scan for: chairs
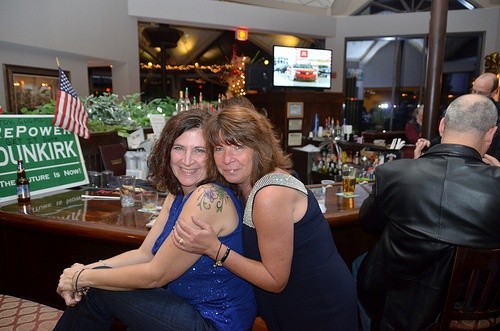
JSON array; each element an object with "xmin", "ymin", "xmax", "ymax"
[{"xmin": 437, "ymin": 246, "xmax": 500, "ymax": 331}]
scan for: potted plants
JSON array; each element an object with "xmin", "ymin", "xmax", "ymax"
[{"xmin": 372, "ymin": 109, "xmax": 387, "ymax": 130}]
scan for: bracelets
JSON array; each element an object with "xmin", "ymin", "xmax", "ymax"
[
  {"xmin": 215, "ymin": 243, "xmax": 222, "ymax": 261},
  {"xmin": 216, "ymin": 248, "xmax": 231, "ymax": 266},
  {"xmin": 76, "ymin": 268, "xmax": 88, "ymax": 295}
]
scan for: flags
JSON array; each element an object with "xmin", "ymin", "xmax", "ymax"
[{"xmin": 52, "ymin": 67, "xmax": 89, "ymax": 139}]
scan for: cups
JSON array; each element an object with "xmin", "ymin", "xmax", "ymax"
[
  {"xmin": 342, "ymin": 167, "xmax": 356, "ymax": 194},
  {"xmin": 140, "ymin": 188, "xmax": 158, "ymax": 219},
  {"xmin": 311, "ymin": 188, "xmax": 325, "ymax": 211},
  {"xmin": 321, "ymin": 180, "xmax": 334, "ymax": 194},
  {"xmin": 119, "ymin": 176, "xmax": 135, "ymax": 207},
  {"xmin": 88, "ymin": 170, "xmax": 113, "ymax": 188}
]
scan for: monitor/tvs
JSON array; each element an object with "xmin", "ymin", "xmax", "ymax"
[{"xmin": 271, "ymin": 45, "xmax": 333, "ymax": 88}]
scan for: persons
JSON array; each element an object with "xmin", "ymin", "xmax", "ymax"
[
  {"xmin": 172, "ymin": 105, "xmax": 360, "ymax": 331},
  {"xmin": 51, "ymin": 109, "xmax": 257, "ymax": 331},
  {"xmin": 403, "ymin": 105, "xmax": 424, "ymax": 159},
  {"xmin": 352, "ymin": 94, "xmax": 500, "ymax": 331},
  {"xmin": 472, "ymin": 73, "xmax": 500, "ymax": 162}
]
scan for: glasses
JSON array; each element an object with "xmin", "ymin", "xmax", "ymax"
[{"xmin": 470, "ymin": 88, "xmax": 495, "ymax": 96}]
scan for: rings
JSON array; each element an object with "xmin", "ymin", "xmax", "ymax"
[{"xmin": 179, "ymin": 239, "xmax": 184, "ymax": 245}]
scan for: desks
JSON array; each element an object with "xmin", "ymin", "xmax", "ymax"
[
  {"xmin": 0, "ymin": 188, "xmax": 166, "ymax": 310},
  {"xmin": 312, "ymin": 183, "xmax": 375, "ymax": 220}
]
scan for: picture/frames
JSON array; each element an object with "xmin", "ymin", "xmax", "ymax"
[{"xmin": 3, "ymin": 64, "xmax": 71, "ymax": 113}]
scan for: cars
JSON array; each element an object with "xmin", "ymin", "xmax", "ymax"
[
  {"xmin": 363, "ymin": 87, "xmax": 419, "ymax": 120},
  {"xmin": 274, "ymin": 63, "xmax": 330, "ymax": 81}
]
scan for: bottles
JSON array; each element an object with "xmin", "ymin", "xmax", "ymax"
[
  {"xmin": 192, "ymin": 93, "xmax": 206, "ymax": 112},
  {"xmin": 335, "ymin": 118, "xmax": 346, "ymax": 138},
  {"xmin": 176, "ymin": 88, "xmax": 191, "ymax": 112},
  {"xmin": 312, "ymin": 144, "xmax": 342, "ymax": 176},
  {"xmin": 17, "ymin": 160, "xmax": 30, "ymax": 203},
  {"xmin": 206, "ymin": 94, "xmax": 226, "ymax": 114},
  {"xmin": 343, "ymin": 150, "xmax": 383, "ymax": 178},
  {"xmin": 324, "ymin": 116, "xmax": 335, "ymax": 139}
]
